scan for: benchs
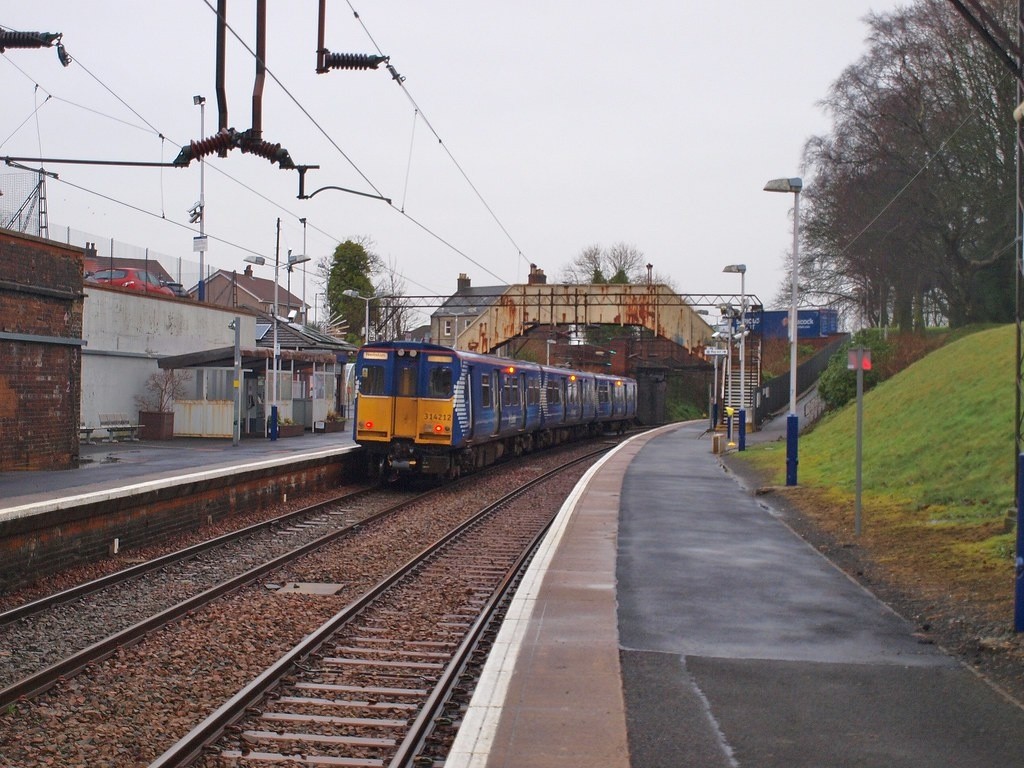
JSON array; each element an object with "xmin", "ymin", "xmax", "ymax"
[
  {"xmin": 80, "ymin": 414, "xmax": 102, "ymax": 445},
  {"xmin": 98, "ymin": 413, "xmax": 146, "ymax": 443}
]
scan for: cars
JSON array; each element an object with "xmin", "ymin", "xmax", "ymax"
[
  {"xmin": 159, "ymin": 280, "xmax": 192, "ymax": 299},
  {"xmin": 88, "ymin": 268, "xmax": 176, "ymax": 297}
]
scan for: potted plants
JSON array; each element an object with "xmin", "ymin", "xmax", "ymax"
[
  {"xmin": 267, "ymin": 417, "xmax": 305, "ymax": 438},
  {"xmin": 314, "ymin": 408, "xmax": 347, "ymax": 433},
  {"xmin": 133, "ymin": 368, "xmax": 194, "ymax": 438}
]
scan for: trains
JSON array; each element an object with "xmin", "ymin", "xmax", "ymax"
[{"xmin": 351, "ymin": 341, "xmax": 638, "ymax": 487}]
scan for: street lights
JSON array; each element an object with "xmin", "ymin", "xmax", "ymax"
[
  {"xmin": 243, "ymin": 254, "xmax": 312, "ymax": 441},
  {"xmin": 722, "ymin": 264, "xmax": 748, "ymax": 452},
  {"xmin": 340, "ymin": 289, "xmax": 395, "ymax": 344},
  {"xmin": 694, "ymin": 308, "xmax": 724, "ymax": 428},
  {"xmin": 762, "ymin": 177, "xmax": 806, "ymax": 488}
]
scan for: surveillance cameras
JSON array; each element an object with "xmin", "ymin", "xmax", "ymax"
[
  {"xmin": 735, "ymin": 331, "xmax": 751, "ymax": 340},
  {"xmin": 288, "ymin": 310, "xmax": 297, "ymax": 322},
  {"xmin": 190, "ymin": 213, "xmax": 200, "ymax": 224},
  {"xmin": 712, "ymin": 332, "xmax": 721, "ymax": 340},
  {"xmin": 187, "ymin": 201, "xmax": 201, "ymax": 213}
]
